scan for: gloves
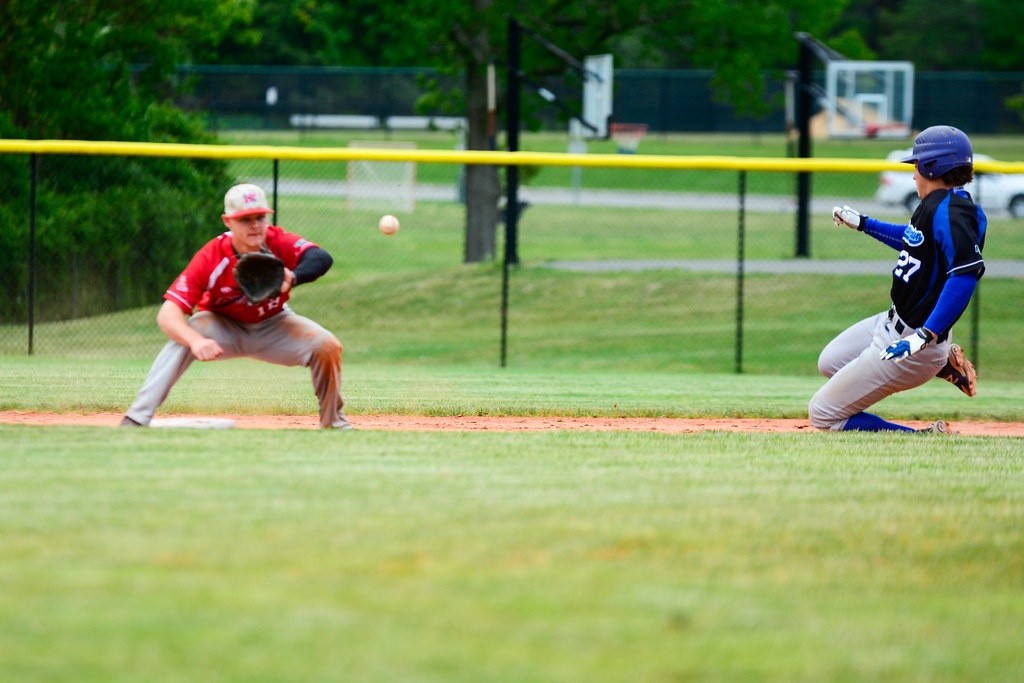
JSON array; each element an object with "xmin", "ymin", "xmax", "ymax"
[
  {"xmin": 880, "ymin": 327, "xmax": 934, "ymax": 364},
  {"xmin": 832, "ymin": 205, "xmax": 868, "ymax": 231}
]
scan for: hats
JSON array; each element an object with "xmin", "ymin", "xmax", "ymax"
[{"xmin": 225, "ymin": 182, "xmax": 274, "ymax": 218}]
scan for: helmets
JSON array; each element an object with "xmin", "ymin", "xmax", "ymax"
[{"xmin": 899, "ymin": 125, "xmax": 973, "ymax": 179}]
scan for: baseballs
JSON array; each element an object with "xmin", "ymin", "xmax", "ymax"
[{"xmin": 379, "ymin": 215, "xmax": 399, "ymax": 235}]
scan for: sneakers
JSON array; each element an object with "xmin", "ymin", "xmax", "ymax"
[
  {"xmin": 930, "ymin": 420, "xmax": 949, "ymax": 435},
  {"xmin": 932, "ymin": 343, "xmax": 977, "ymax": 397}
]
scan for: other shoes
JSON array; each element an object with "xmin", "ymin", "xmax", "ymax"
[
  {"xmin": 324, "ymin": 419, "xmax": 357, "ymax": 429},
  {"xmin": 121, "ymin": 417, "xmax": 137, "ymax": 427}
]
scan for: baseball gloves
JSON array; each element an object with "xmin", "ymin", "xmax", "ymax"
[{"xmin": 233, "ymin": 252, "xmax": 284, "ymax": 302}]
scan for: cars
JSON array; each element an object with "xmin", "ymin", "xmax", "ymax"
[{"xmin": 874, "ymin": 147, "xmax": 1023, "ymax": 222}]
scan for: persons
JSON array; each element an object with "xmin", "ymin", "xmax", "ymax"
[
  {"xmin": 120, "ymin": 183, "xmax": 358, "ymax": 432},
  {"xmin": 808, "ymin": 125, "xmax": 988, "ymax": 434}
]
